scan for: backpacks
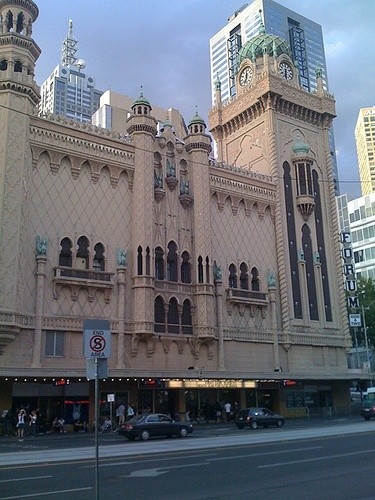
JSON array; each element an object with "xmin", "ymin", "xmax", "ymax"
[{"xmin": 130, "ymin": 406, "xmax": 137, "ymax": 414}]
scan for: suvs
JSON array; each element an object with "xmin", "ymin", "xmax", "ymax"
[{"xmin": 234, "ymin": 407, "xmax": 286, "ymax": 431}]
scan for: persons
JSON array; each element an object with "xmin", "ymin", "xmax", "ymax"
[
  {"xmin": 52, "ymin": 417, "xmax": 65, "ymax": 433},
  {"xmin": 0, "ymin": 407, "xmax": 38, "ymax": 438},
  {"xmin": 115, "ymin": 403, "xmax": 151, "ymax": 426},
  {"xmin": 204, "ymin": 401, "xmax": 240, "ymax": 423},
  {"xmin": 93, "ymin": 416, "xmax": 112, "ymax": 432},
  {"xmin": 74, "ymin": 419, "xmax": 87, "ymax": 432}
]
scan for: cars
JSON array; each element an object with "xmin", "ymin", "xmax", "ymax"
[
  {"xmin": 351, "ymin": 387, "xmax": 375, "ymax": 421},
  {"xmin": 119, "ymin": 413, "xmax": 194, "ymax": 441}
]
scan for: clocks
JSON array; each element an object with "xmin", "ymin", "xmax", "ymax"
[
  {"xmin": 239, "ymin": 64, "xmax": 254, "ymax": 88},
  {"xmin": 280, "ymin": 62, "xmax": 294, "ymax": 80}
]
